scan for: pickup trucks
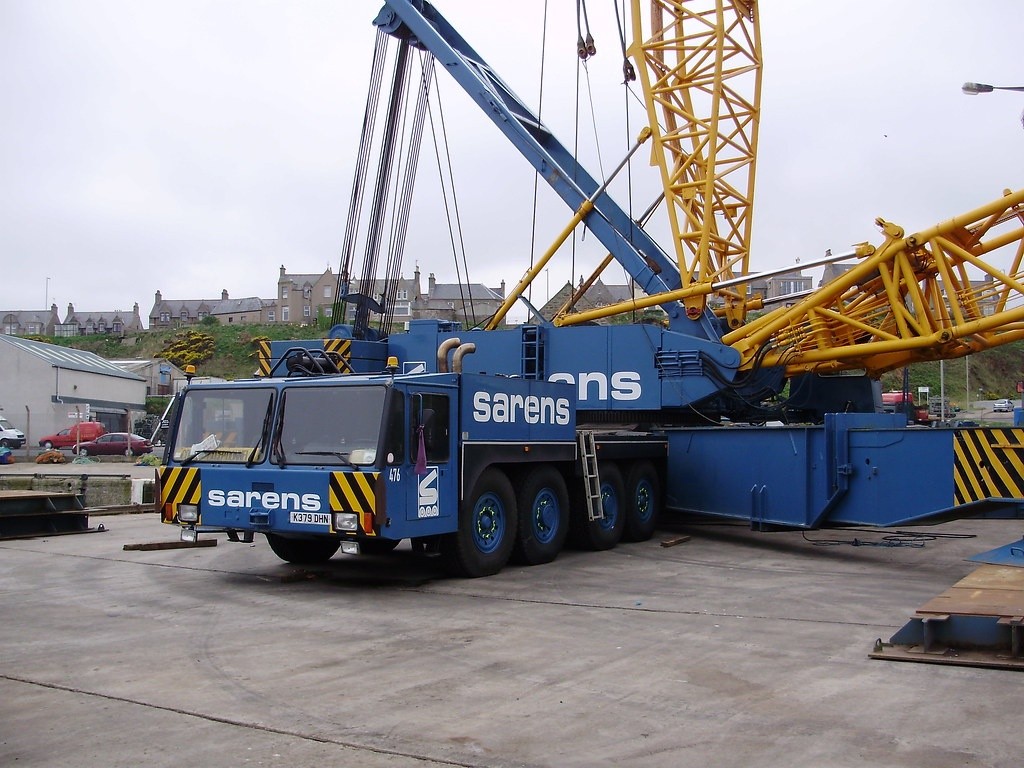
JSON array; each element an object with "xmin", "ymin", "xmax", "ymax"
[{"xmin": 39, "ymin": 422, "xmax": 105, "ymax": 450}]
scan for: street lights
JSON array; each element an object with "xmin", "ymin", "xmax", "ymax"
[
  {"xmin": 545, "ymin": 268, "xmax": 548, "ymax": 302},
  {"xmin": 44, "ymin": 277, "xmax": 51, "ymax": 337}
]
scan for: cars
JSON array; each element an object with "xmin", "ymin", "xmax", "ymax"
[
  {"xmin": 992, "ymin": 398, "xmax": 1015, "ymax": 412},
  {"xmin": 72, "ymin": 432, "xmax": 154, "ymax": 458}
]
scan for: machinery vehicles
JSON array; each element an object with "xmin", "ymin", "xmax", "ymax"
[{"xmin": 142, "ymin": 0, "xmax": 1024, "ymax": 584}]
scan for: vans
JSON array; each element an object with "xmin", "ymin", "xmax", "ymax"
[{"xmin": 0, "ymin": 414, "xmax": 26, "ymax": 449}]
